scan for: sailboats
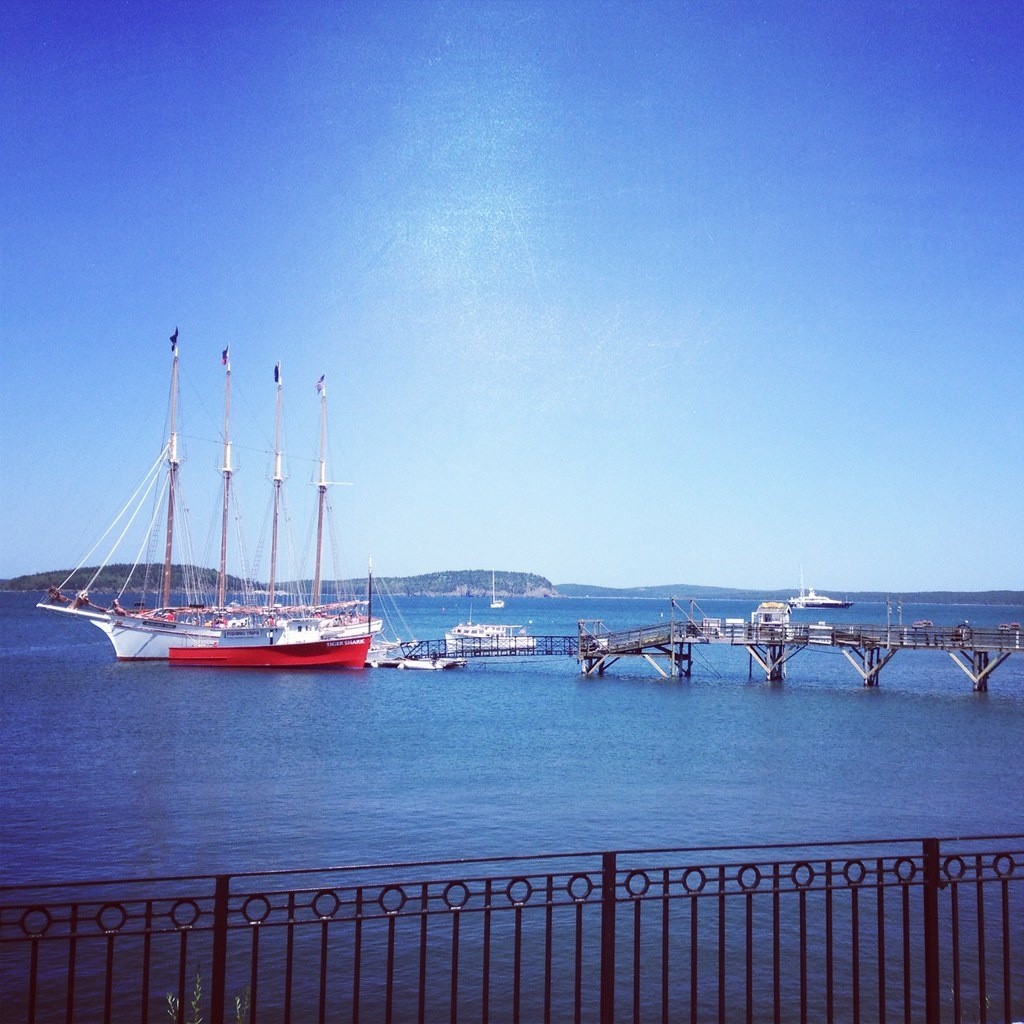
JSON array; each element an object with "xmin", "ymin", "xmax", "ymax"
[
  {"xmin": 359, "ymin": 554, "xmax": 418, "ymax": 667},
  {"xmin": 37, "ymin": 328, "xmax": 383, "ymax": 665},
  {"xmin": 444, "ymin": 603, "xmax": 536, "ymax": 653},
  {"xmin": 787, "ymin": 563, "xmax": 855, "ymax": 609},
  {"xmin": 489, "ymin": 569, "xmax": 505, "ymax": 609}
]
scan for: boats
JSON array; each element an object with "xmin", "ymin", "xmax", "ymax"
[
  {"xmin": 1009, "ymin": 623, "xmax": 1021, "ymax": 630},
  {"xmin": 168, "ymin": 634, "xmax": 372, "ymax": 669},
  {"xmin": 134, "ymin": 597, "xmax": 147, "ymax": 606},
  {"xmin": 923, "ymin": 620, "xmax": 933, "ymax": 627},
  {"xmin": 378, "ymin": 655, "xmax": 467, "ymax": 669},
  {"xmin": 998, "ymin": 624, "xmax": 1011, "ymax": 631},
  {"xmin": 912, "ymin": 621, "xmax": 924, "ymax": 629}
]
